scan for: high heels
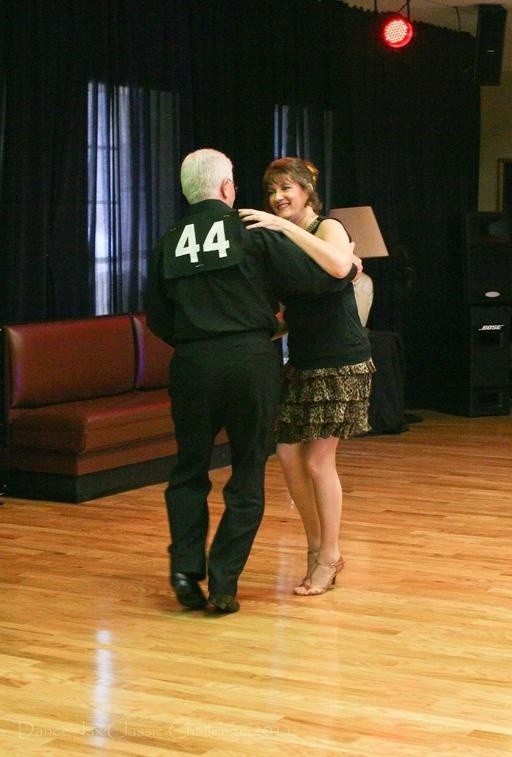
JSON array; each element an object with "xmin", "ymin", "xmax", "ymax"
[{"xmin": 293, "ymin": 551, "xmax": 344, "ymax": 594}]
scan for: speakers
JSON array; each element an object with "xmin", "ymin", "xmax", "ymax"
[{"xmin": 472, "ymin": 3, "xmax": 508, "ymax": 86}]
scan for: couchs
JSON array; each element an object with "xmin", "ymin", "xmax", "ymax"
[{"xmin": 1, "ymin": 311, "xmax": 280, "ymax": 505}]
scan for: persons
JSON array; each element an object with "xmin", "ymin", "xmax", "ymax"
[
  {"xmin": 226, "ymin": 157, "xmax": 359, "ymax": 598},
  {"xmin": 140, "ymin": 147, "xmax": 366, "ymax": 613}
]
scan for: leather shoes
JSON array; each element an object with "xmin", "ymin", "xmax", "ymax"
[
  {"xmin": 206, "ymin": 596, "xmax": 242, "ymax": 612},
  {"xmin": 168, "ymin": 573, "xmax": 208, "ymax": 608}
]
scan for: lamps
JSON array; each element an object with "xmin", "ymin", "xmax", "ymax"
[
  {"xmin": 370, "ymin": 1, "xmax": 416, "ymax": 53},
  {"xmin": 327, "ymin": 204, "xmax": 389, "ymax": 330}
]
